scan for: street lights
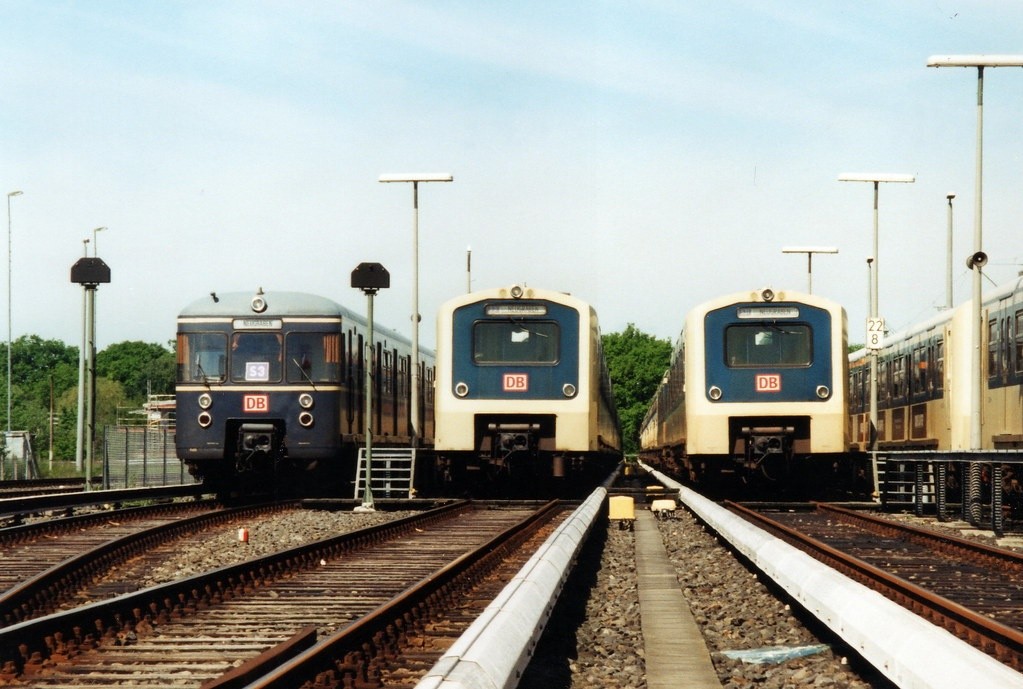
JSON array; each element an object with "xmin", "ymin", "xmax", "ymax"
[
  {"xmin": 86, "ymin": 225, "xmax": 108, "ymax": 490},
  {"xmin": 379, "ymin": 174, "xmax": 454, "ymax": 447},
  {"xmin": 782, "ymin": 247, "xmax": 839, "ymax": 297},
  {"xmin": 839, "ymin": 175, "xmax": 915, "ymax": 451},
  {"xmin": 865, "ymin": 256, "xmax": 874, "ymax": 318},
  {"xmin": 926, "ymin": 51, "xmax": 1023, "ymax": 448},
  {"xmin": 945, "ymin": 193, "xmax": 956, "ymax": 312},
  {"xmin": 7, "ymin": 190, "xmax": 24, "ymax": 433}
]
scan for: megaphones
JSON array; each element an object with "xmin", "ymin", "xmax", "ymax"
[
  {"xmin": 972, "ymin": 252, "xmax": 989, "ymax": 267},
  {"xmin": 966, "ymin": 255, "xmax": 974, "ymax": 269}
]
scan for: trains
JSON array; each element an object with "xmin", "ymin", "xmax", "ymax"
[
  {"xmin": 638, "ymin": 288, "xmax": 845, "ymax": 498},
  {"xmin": 847, "ymin": 272, "xmax": 1023, "ymax": 527},
  {"xmin": 174, "ymin": 286, "xmax": 435, "ymax": 502},
  {"xmin": 434, "ymin": 283, "xmax": 625, "ymax": 503}
]
